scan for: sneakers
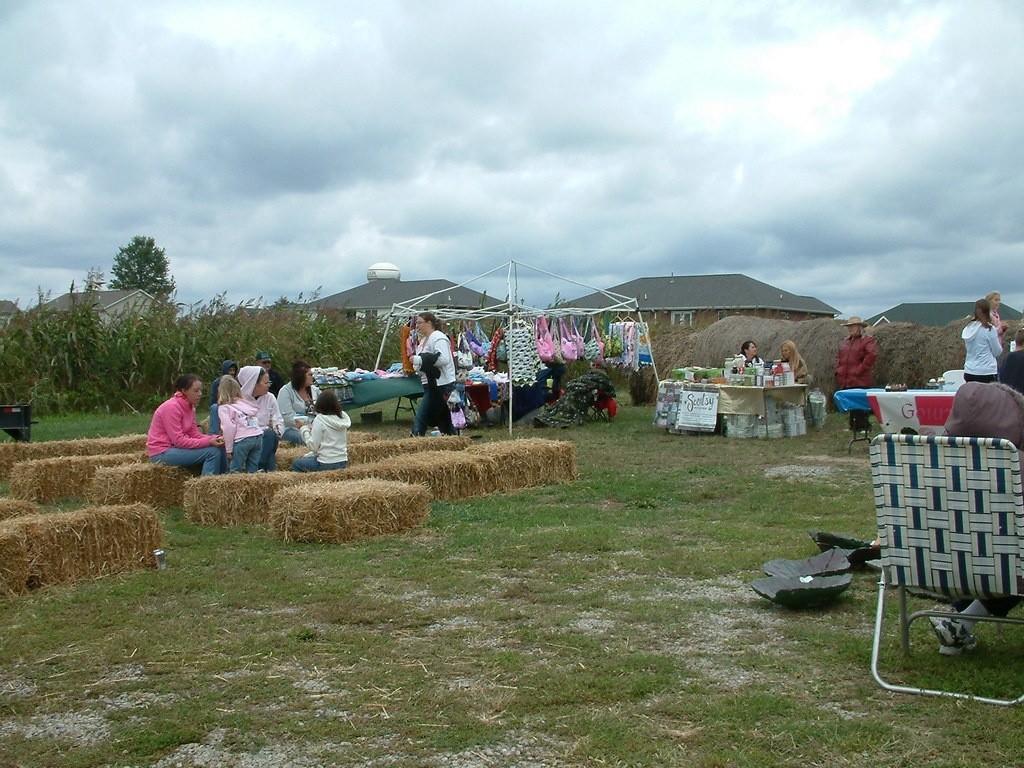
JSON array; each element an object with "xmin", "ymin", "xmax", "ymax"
[
  {"xmin": 928, "ymin": 606, "xmax": 968, "ymax": 647},
  {"xmin": 938, "ymin": 631, "xmax": 978, "ymax": 657}
]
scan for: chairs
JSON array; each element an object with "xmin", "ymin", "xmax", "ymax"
[
  {"xmin": 943, "ymin": 369, "xmax": 966, "ymax": 392},
  {"xmin": 870, "ymin": 433, "xmax": 1024, "ymax": 705},
  {"xmin": 803, "ymin": 374, "xmax": 816, "ymax": 425}
]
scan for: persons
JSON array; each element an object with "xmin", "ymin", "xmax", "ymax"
[
  {"xmin": 209, "ymin": 356, "xmax": 239, "ymax": 435},
  {"xmin": 146, "ymin": 373, "xmax": 230, "ymax": 476},
  {"xmin": 409, "ymin": 313, "xmax": 460, "ymax": 437},
  {"xmin": 276, "ymin": 360, "xmax": 324, "ymax": 444},
  {"xmin": 732, "ymin": 340, "xmax": 767, "ymax": 370},
  {"xmin": 871, "ymin": 383, "xmax": 1024, "ymax": 656},
  {"xmin": 780, "ymin": 338, "xmax": 810, "ymax": 384},
  {"xmin": 291, "ymin": 390, "xmax": 353, "ymax": 472},
  {"xmin": 235, "ymin": 366, "xmax": 286, "ymax": 474},
  {"xmin": 253, "ymin": 351, "xmax": 285, "ymax": 400},
  {"xmin": 834, "ymin": 317, "xmax": 880, "ymax": 433},
  {"xmin": 961, "ymin": 298, "xmax": 1003, "ymax": 385},
  {"xmin": 218, "ymin": 374, "xmax": 263, "ymax": 474},
  {"xmin": 985, "ymin": 291, "xmax": 1010, "ymax": 351},
  {"xmin": 1001, "ymin": 327, "xmax": 1024, "ymax": 395}
]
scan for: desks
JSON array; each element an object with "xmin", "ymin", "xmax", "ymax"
[
  {"xmin": 652, "ymin": 377, "xmax": 808, "ymax": 442},
  {"xmin": 834, "ymin": 387, "xmax": 956, "ymax": 455},
  {"xmin": 314, "ymin": 373, "xmax": 509, "ymax": 438}
]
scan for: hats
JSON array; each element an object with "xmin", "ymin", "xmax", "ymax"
[
  {"xmin": 255, "ymin": 350, "xmax": 272, "ymax": 361},
  {"xmin": 840, "ymin": 316, "xmax": 868, "ymax": 328}
]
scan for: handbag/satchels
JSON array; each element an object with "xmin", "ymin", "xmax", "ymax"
[{"xmin": 447, "ymin": 311, "xmax": 638, "ymax": 370}]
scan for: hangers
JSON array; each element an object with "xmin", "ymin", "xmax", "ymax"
[
  {"xmin": 618, "ymin": 310, "xmax": 637, "ymax": 326},
  {"xmin": 609, "ymin": 309, "xmax": 624, "ymax": 326},
  {"xmin": 503, "ymin": 313, "xmax": 534, "ymax": 330}
]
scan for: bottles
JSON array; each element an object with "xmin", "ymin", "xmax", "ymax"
[
  {"xmin": 810, "ymin": 386, "xmax": 826, "ymax": 426},
  {"xmin": 724, "ymin": 356, "xmax": 794, "ymax": 387}
]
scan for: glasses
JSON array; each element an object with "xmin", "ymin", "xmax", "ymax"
[
  {"xmin": 415, "ymin": 321, "xmax": 423, "ymax": 324},
  {"xmin": 260, "ymin": 360, "xmax": 272, "ymax": 365}
]
